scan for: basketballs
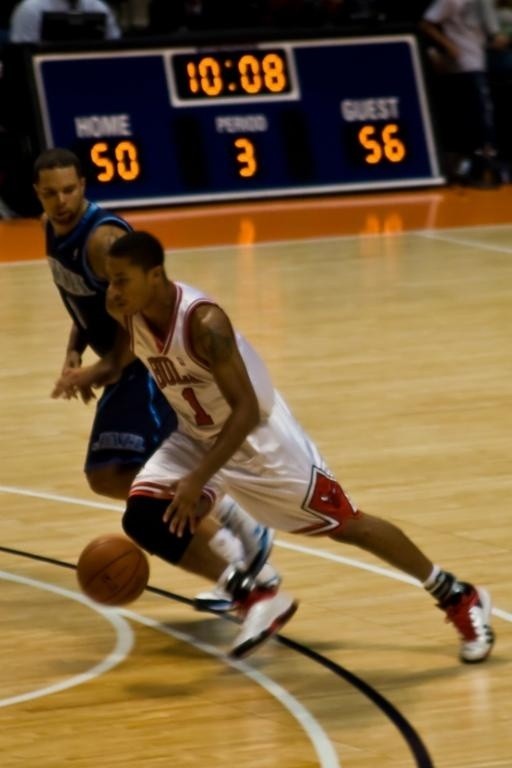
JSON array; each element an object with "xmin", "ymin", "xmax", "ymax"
[{"xmin": 76, "ymin": 536, "xmax": 149, "ymax": 604}]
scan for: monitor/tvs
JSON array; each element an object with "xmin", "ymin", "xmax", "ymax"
[{"xmin": 41, "ymin": 10, "xmax": 108, "ymax": 41}]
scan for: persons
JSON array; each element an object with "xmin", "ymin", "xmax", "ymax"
[
  {"xmin": 104, "ymin": 229, "xmax": 497, "ymax": 663},
  {"xmin": 8, "ymin": 0, "xmax": 123, "ymax": 43},
  {"xmin": 30, "ymin": 150, "xmax": 281, "ymax": 616},
  {"xmin": 420, "ymin": 0, "xmax": 512, "ymax": 157}
]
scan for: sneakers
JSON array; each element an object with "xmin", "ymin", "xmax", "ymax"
[
  {"xmin": 194, "ymin": 523, "xmax": 298, "ymax": 658},
  {"xmin": 435, "ymin": 581, "xmax": 493, "ymax": 662}
]
